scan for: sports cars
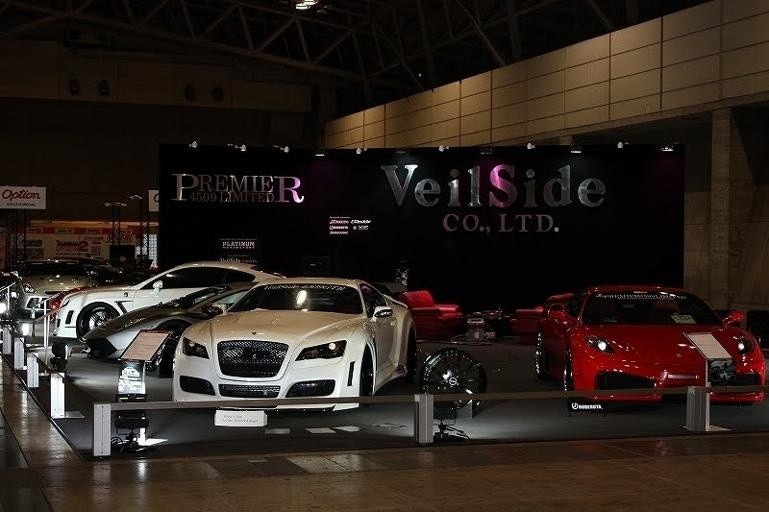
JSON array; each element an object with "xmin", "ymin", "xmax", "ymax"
[
  {"xmin": 1, "ymin": 254, "xmax": 288, "ymax": 365},
  {"xmin": 172, "ymin": 275, "xmax": 420, "ymax": 414},
  {"xmin": 533, "ymin": 275, "xmax": 767, "ymax": 404}
]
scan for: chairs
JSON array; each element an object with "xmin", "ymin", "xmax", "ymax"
[
  {"xmin": 511, "ymin": 293, "xmax": 575, "ymax": 338},
  {"xmin": 399, "ymin": 291, "xmax": 464, "ymax": 341}
]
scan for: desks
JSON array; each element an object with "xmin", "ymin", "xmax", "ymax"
[{"xmin": 457, "ymin": 308, "xmax": 511, "ymax": 337}]
